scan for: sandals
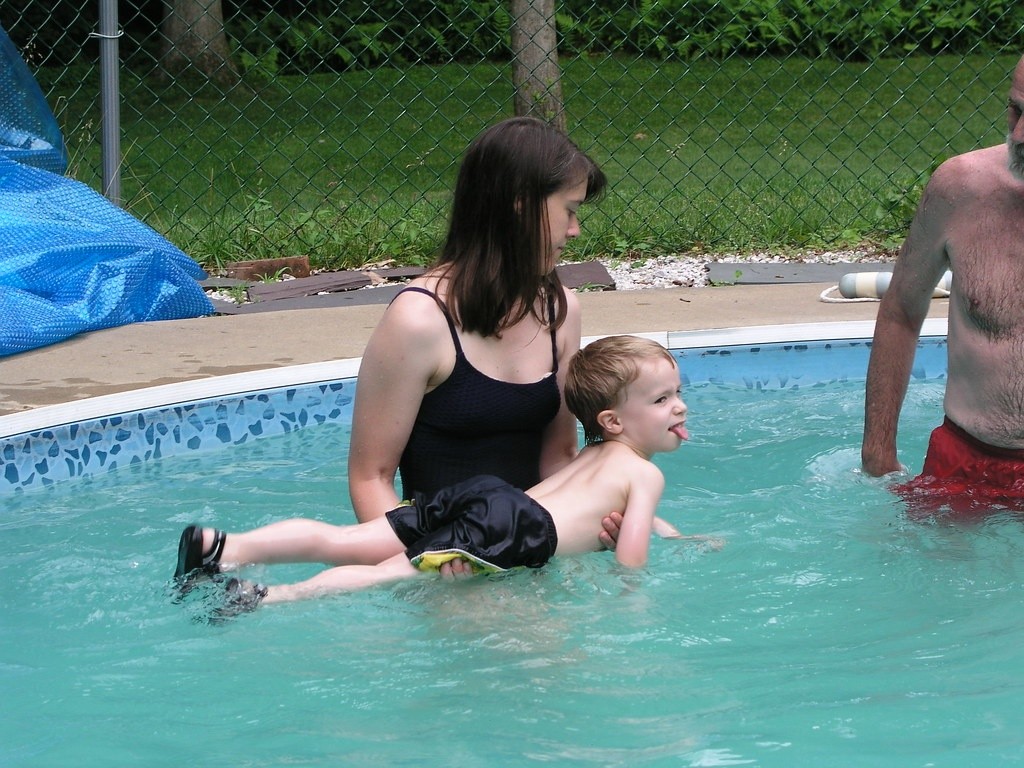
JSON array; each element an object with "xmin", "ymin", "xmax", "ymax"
[
  {"xmin": 171, "ymin": 525, "xmax": 226, "ymax": 581},
  {"xmin": 223, "ymin": 578, "xmax": 269, "ymax": 619}
]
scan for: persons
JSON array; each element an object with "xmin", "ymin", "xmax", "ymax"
[
  {"xmin": 171, "ymin": 336, "xmax": 692, "ymax": 604},
  {"xmin": 861, "ymin": 51, "xmax": 1024, "ymax": 528},
  {"xmin": 346, "ymin": 115, "xmax": 681, "ymax": 584}
]
